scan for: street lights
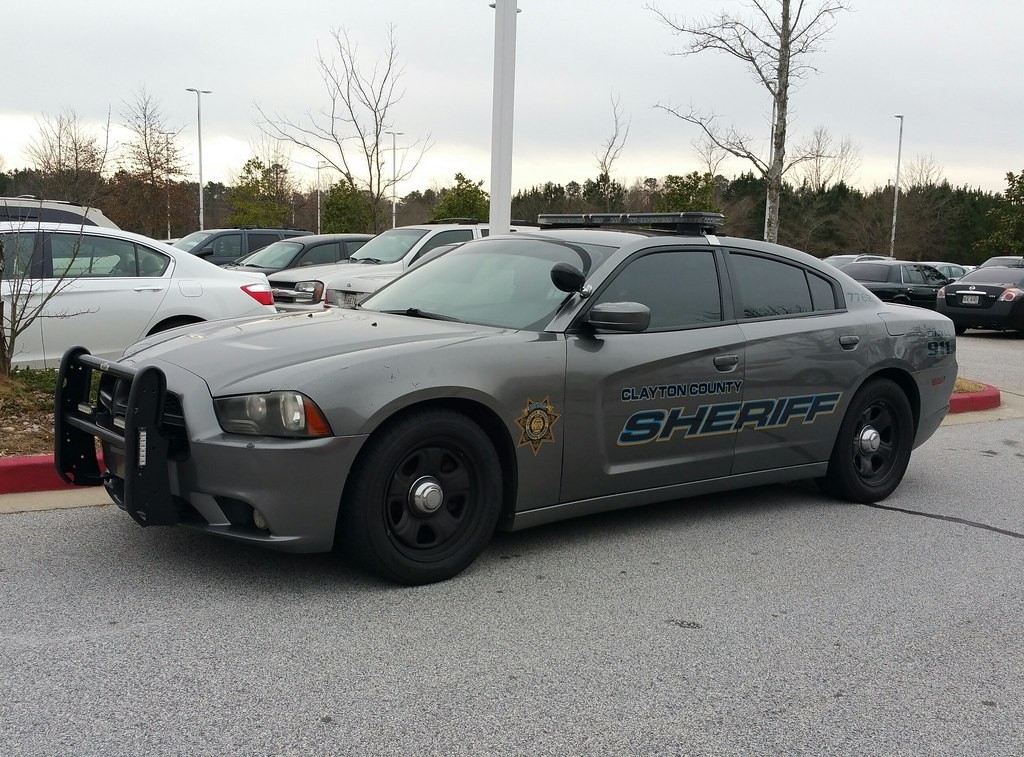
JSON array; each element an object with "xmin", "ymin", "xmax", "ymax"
[
  {"xmin": 889, "ymin": 112, "xmax": 905, "ymax": 257},
  {"xmin": 160, "ymin": 130, "xmax": 177, "ymax": 239},
  {"xmin": 316, "ymin": 161, "xmax": 326, "ymax": 234},
  {"xmin": 386, "ymin": 130, "xmax": 406, "ymax": 228},
  {"xmin": 186, "ymin": 85, "xmax": 212, "ymax": 230}
]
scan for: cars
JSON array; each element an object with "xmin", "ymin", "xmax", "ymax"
[
  {"xmin": 51, "ymin": 206, "xmax": 959, "ymax": 591},
  {"xmin": 220, "ymin": 233, "xmax": 378, "ymax": 278},
  {"xmin": 839, "ymin": 261, "xmax": 956, "ymax": 306},
  {"xmin": 978, "ymin": 256, "xmax": 1024, "ymax": 268},
  {"xmin": 172, "ymin": 225, "xmax": 315, "ymax": 267},
  {"xmin": 923, "ymin": 261, "xmax": 971, "ymax": 282},
  {"xmin": 937, "ymin": 266, "xmax": 1024, "ymax": 336},
  {"xmin": 0, "ymin": 219, "xmax": 278, "ymax": 369}
]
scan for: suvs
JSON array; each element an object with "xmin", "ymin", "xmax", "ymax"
[
  {"xmin": 267, "ymin": 213, "xmax": 543, "ymax": 310},
  {"xmin": 0, "ymin": 194, "xmax": 123, "ymax": 231},
  {"xmin": 823, "ymin": 252, "xmax": 897, "ymax": 271}
]
